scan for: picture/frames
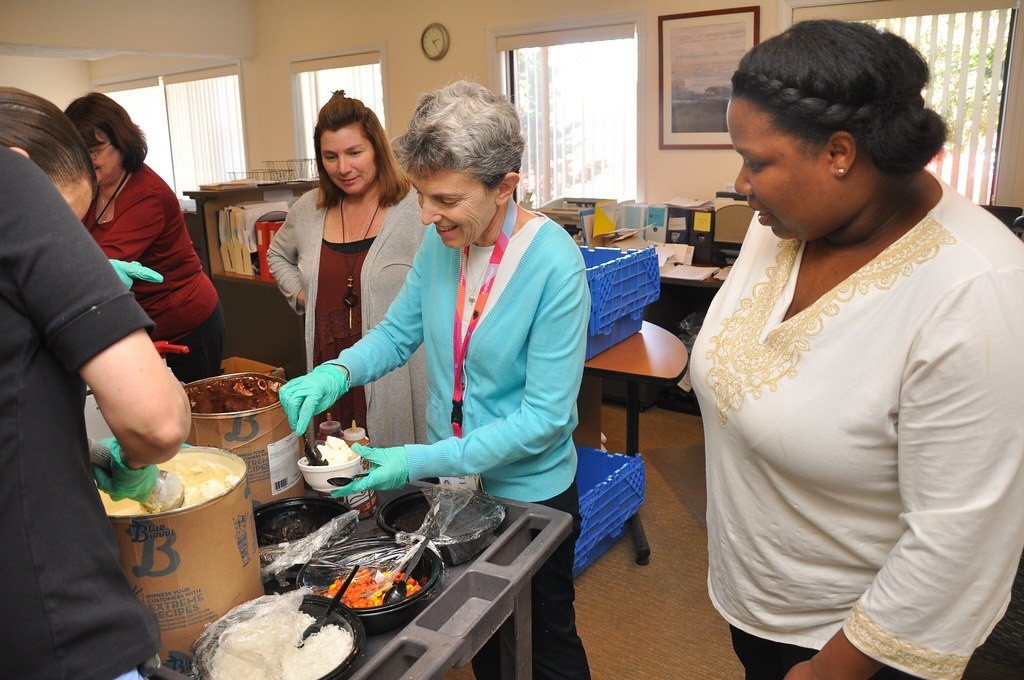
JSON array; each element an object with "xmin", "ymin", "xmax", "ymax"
[{"xmin": 656, "ymin": 4, "xmax": 760, "ymax": 151}]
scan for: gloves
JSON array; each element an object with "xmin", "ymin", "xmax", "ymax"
[
  {"xmin": 279, "ymin": 364, "xmax": 350, "ymax": 437},
  {"xmin": 331, "ymin": 443, "xmax": 408, "ymax": 498},
  {"xmin": 109, "ymin": 259, "xmax": 163, "ymax": 290},
  {"xmin": 92, "ymin": 437, "xmax": 160, "ymax": 504}
]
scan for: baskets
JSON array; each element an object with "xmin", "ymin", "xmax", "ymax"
[{"xmin": 226, "ymin": 158, "xmax": 319, "ymax": 184}]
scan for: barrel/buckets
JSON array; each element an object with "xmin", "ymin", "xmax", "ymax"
[
  {"xmin": 108, "ymin": 446, "xmax": 266, "ymax": 677},
  {"xmin": 183, "ymin": 372, "xmax": 305, "ymax": 504}
]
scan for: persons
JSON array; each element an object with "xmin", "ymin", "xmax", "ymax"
[
  {"xmin": 61, "ymin": 90, "xmax": 226, "ymax": 382},
  {"xmin": 0, "ymin": 84, "xmax": 191, "ymax": 680},
  {"xmin": 277, "ymin": 80, "xmax": 594, "ymax": 680},
  {"xmin": 689, "ymin": 18, "xmax": 1024, "ymax": 680},
  {"xmin": 266, "ymin": 89, "xmax": 435, "ymax": 448}
]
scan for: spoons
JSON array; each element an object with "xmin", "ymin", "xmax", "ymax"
[
  {"xmin": 383, "ymin": 534, "xmax": 430, "ymax": 606},
  {"xmin": 296, "ymin": 564, "xmax": 360, "ymax": 648},
  {"xmin": 327, "ymin": 473, "xmax": 370, "ymax": 488},
  {"xmin": 349, "ymin": 487, "xmax": 350, "ymax": 488}
]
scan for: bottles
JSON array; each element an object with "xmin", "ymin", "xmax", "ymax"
[{"xmin": 316, "ymin": 413, "xmax": 378, "ymax": 520}]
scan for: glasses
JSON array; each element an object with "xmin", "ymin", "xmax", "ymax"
[{"xmin": 89, "ymin": 142, "xmax": 112, "ymax": 160}]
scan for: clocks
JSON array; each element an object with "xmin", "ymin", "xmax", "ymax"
[{"xmin": 420, "ymin": 22, "xmax": 450, "ymax": 61}]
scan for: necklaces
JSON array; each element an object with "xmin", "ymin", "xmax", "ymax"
[
  {"xmin": 84, "ymin": 170, "xmax": 129, "ymax": 233},
  {"xmin": 465, "ymin": 259, "xmax": 488, "ymax": 305},
  {"xmin": 100, "ymin": 199, "xmax": 109, "ymax": 223},
  {"xmin": 340, "ymin": 195, "xmax": 379, "ymax": 329}
]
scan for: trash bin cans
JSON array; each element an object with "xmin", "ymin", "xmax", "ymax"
[{"xmin": 670, "ymin": 310, "xmax": 710, "ymax": 414}]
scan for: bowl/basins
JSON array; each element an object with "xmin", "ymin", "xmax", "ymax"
[
  {"xmin": 296, "ymin": 536, "xmax": 442, "ymax": 635},
  {"xmin": 296, "ymin": 454, "xmax": 361, "ymax": 493},
  {"xmin": 375, "ymin": 486, "xmax": 506, "ymax": 568},
  {"xmin": 252, "ymin": 495, "xmax": 359, "ymax": 582},
  {"xmin": 191, "ymin": 595, "xmax": 367, "ymax": 679}
]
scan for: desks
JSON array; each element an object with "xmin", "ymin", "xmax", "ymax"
[
  {"xmin": 184, "ymin": 178, "xmax": 320, "ymax": 382},
  {"xmin": 575, "ymin": 322, "xmax": 689, "ymax": 567},
  {"xmin": 632, "ymin": 258, "xmax": 732, "ymax": 413}
]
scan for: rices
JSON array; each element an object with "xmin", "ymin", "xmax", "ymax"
[
  {"xmin": 208, "ymin": 610, "xmax": 353, "ymax": 680},
  {"xmin": 302, "ymin": 435, "xmax": 355, "ymax": 468}
]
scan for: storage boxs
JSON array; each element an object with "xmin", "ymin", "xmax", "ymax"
[
  {"xmin": 568, "ymin": 447, "xmax": 644, "ymax": 578},
  {"xmin": 580, "ymin": 246, "xmax": 660, "ymax": 362}
]
